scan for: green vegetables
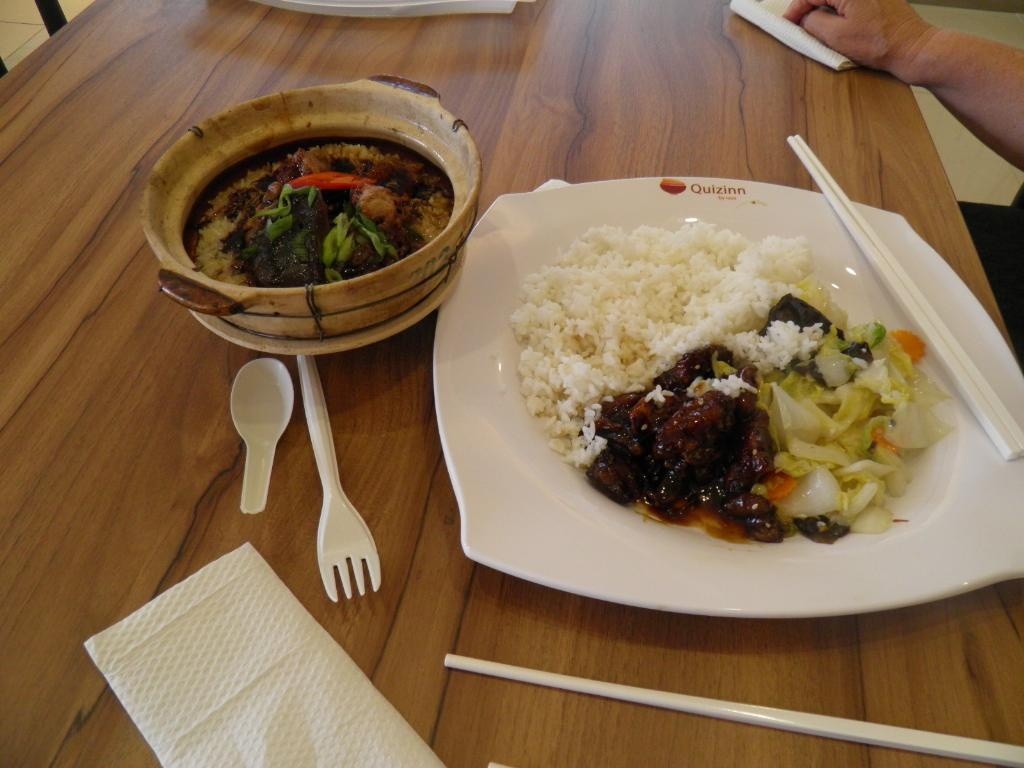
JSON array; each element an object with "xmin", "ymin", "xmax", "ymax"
[{"xmin": 246, "ymin": 184, "xmax": 398, "ymax": 283}]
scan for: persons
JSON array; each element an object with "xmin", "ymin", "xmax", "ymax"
[{"xmin": 780, "ymin": 0, "xmax": 1023, "ymax": 375}]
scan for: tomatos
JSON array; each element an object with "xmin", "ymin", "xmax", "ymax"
[{"xmin": 291, "ymin": 170, "xmax": 376, "ymax": 191}]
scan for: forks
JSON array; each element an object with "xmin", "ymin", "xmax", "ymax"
[{"xmin": 289, "ymin": 355, "xmax": 382, "ymax": 604}]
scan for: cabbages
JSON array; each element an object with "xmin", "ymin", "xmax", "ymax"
[{"xmin": 759, "ymin": 321, "xmax": 958, "ymax": 536}]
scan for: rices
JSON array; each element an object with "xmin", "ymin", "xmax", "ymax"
[{"xmin": 510, "ymin": 221, "xmax": 814, "ymax": 465}]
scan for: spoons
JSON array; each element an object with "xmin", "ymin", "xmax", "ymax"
[{"xmin": 227, "ymin": 358, "xmax": 294, "ymax": 515}]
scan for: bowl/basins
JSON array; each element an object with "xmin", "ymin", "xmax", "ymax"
[{"xmin": 144, "ymin": 74, "xmax": 480, "ymax": 356}]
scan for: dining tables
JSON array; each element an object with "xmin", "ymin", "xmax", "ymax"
[{"xmin": 1, "ymin": 2, "xmax": 1024, "ymax": 768}]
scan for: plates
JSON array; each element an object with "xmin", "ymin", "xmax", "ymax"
[{"xmin": 434, "ymin": 168, "xmax": 1023, "ymax": 625}]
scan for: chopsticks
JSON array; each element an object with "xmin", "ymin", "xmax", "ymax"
[{"xmin": 787, "ymin": 130, "xmax": 1023, "ymax": 464}]
